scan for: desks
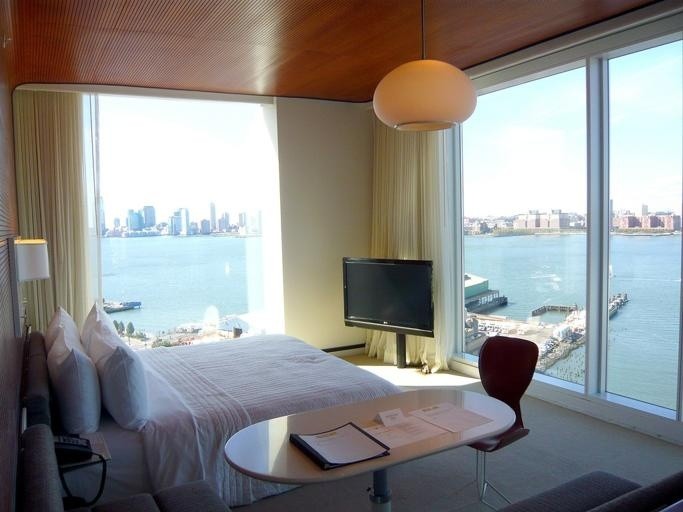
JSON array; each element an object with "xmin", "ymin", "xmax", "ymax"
[{"xmin": 222, "ymin": 387, "xmax": 517, "ymax": 512}]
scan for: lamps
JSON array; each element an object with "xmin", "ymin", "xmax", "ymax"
[
  {"xmin": 14, "ymin": 238, "xmax": 50, "ymax": 281},
  {"xmin": 372, "ymin": 0, "xmax": 477, "ymax": 133}
]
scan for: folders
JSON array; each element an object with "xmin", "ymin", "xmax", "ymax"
[{"xmin": 288, "ymin": 422, "xmax": 391, "ymax": 470}]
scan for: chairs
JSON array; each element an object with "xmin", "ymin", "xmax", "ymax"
[
  {"xmin": 498, "ymin": 470, "xmax": 683, "ymax": 512},
  {"xmin": 462, "ymin": 335, "xmax": 538, "ymax": 512},
  {"xmin": 23, "ymin": 424, "xmax": 230, "ymax": 508}
]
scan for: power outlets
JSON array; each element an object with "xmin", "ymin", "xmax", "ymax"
[{"xmin": 20, "ymin": 408, "xmax": 27, "ymax": 434}]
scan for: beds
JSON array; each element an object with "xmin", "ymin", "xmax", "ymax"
[{"xmin": 22, "ymin": 331, "xmax": 402, "ymax": 509}]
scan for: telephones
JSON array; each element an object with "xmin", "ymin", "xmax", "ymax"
[{"xmin": 51, "ymin": 429, "xmax": 91, "ymax": 463}]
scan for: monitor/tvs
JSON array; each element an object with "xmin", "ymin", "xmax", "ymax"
[{"xmin": 342, "ymin": 257, "xmax": 437, "ymax": 338}]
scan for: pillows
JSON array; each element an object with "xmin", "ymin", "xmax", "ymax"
[
  {"xmin": 46, "ymin": 330, "xmax": 100, "ymax": 434},
  {"xmin": 81, "ymin": 300, "xmax": 119, "ymax": 346},
  {"xmin": 45, "ymin": 307, "xmax": 81, "ymax": 349},
  {"xmin": 88, "ymin": 321, "xmax": 150, "ymax": 432}
]
